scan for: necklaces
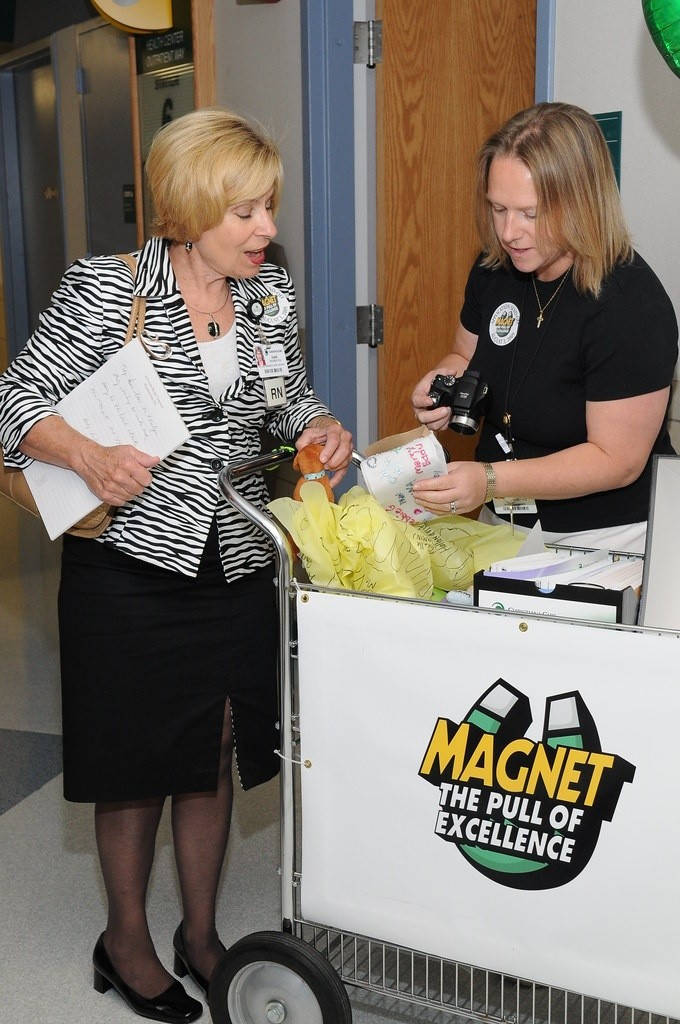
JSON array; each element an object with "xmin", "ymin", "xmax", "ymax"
[
  {"xmin": 185, "ymin": 284, "xmax": 229, "ymax": 337},
  {"xmin": 532, "ymin": 258, "xmax": 575, "ymax": 328}
]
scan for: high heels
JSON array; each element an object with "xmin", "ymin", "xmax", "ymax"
[
  {"xmin": 93, "ymin": 926, "xmax": 203, "ymax": 1024},
  {"xmin": 172, "ymin": 919, "xmax": 227, "ymax": 1006}
]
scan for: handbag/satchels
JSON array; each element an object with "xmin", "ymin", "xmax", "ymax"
[{"xmin": 0, "ymin": 253, "xmax": 146, "ymax": 538}]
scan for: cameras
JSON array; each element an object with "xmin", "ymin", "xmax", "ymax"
[{"xmin": 424, "ymin": 369, "xmax": 495, "ymax": 436}]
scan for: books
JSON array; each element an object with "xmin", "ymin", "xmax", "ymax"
[{"xmin": 488, "ymin": 553, "xmax": 644, "ymax": 597}]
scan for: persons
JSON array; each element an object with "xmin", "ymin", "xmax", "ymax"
[
  {"xmin": 0, "ymin": 110, "xmax": 354, "ymax": 1024},
  {"xmin": 409, "ymin": 102, "xmax": 679, "ymax": 559}
]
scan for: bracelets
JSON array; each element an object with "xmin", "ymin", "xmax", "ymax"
[
  {"xmin": 482, "ymin": 461, "xmax": 495, "ymax": 503},
  {"xmin": 316, "ymin": 417, "xmax": 341, "ymax": 427}
]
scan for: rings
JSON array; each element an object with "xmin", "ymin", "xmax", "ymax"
[
  {"xmin": 415, "ymin": 408, "xmax": 421, "ymax": 420},
  {"xmin": 451, "ymin": 502, "xmax": 455, "ymax": 513}
]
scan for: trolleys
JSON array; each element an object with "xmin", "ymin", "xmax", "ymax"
[{"xmin": 205, "ymin": 446, "xmax": 680, "ymax": 1024}]
